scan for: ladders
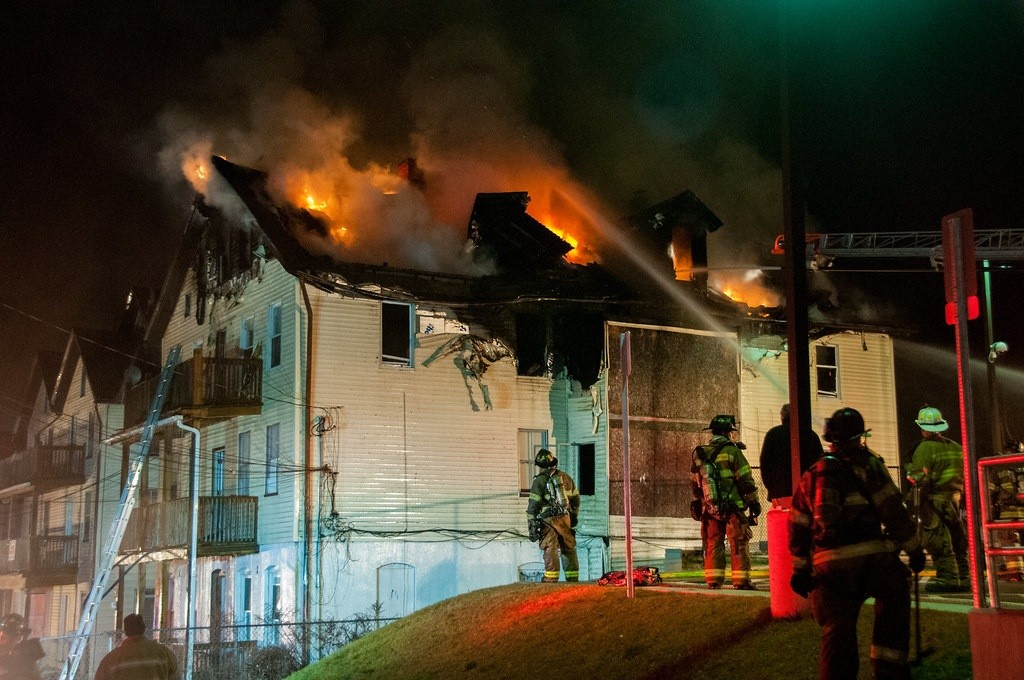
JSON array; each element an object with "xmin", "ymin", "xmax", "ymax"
[{"xmin": 58, "ymin": 344, "xmax": 183, "ymax": 680}]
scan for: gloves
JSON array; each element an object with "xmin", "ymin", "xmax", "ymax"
[
  {"xmin": 747, "ymin": 500, "xmax": 761, "ymax": 518},
  {"xmin": 790, "ymin": 568, "xmax": 814, "ymax": 598},
  {"xmin": 570, "ymin": 513, "xmax": 578, "ymax": 527},
  {"xmin": 907, "ymin": 544, "xmax": 926, "ymax": 574}
]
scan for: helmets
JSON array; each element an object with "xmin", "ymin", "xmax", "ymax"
[
  {"xmin": 823, "ymin": 407, "xmax": 865, "ymax": 442},
  {"xmin": 915, "ymin": 406, "xmax": 949, "ymax": 432},
  {"xmin": 535, "ymin": 449, "xmax": 557, "ymax": 468},
  {"xmin": 0, "ymin": 613, "xmax": 31, "ymax": 636},
  {"xmin": 703, "ymin": 416, "xmax": 738, "ymax": 430}
]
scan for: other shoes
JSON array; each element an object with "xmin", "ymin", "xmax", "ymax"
[
  {"xmin": 542, "ymin": 577, "xmax": 558, "ymax": 583},
  {"xmin": 871, "ymin": 660, "xmax": 912, "ymax": 680},
  {"xmin": 733, "ymin": 581, "xmax": 758, "ymax": 590},
  {"xmin": 925, "ymin": 583, "xmax": 970, "ymax": 594},
  {"xmin": 708, "ymin": 583, "xmax": 721, "ymax": 589},
  {"xmin": 566, "ymin": 578, "xmax": 578, "ymax": 582}
]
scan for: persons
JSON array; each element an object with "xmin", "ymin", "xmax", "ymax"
[
  {"xmin": 787, "ymin": 407, "xmax": 928, "ymax": 680},
  {"xmin": 93, "ymin": 613, "xmax": 181, "ymax": 680},
  {"xmin": 757, "ymin": 404, "xmax": 826, "ymax": 503},
  {"xmin": 686, "ymin": 415, "xmax": 763, "ymax": 590},
  {"xmin": 526, "ymin": 449, "xmax": 582, "ymax": 583},
  {"xmin": 0, "ymin": 612, "xmax": 46, "ymax": 680},
  {"xmin": 907, "ymin": 402, "xmax": 974, "ymax": 595}
]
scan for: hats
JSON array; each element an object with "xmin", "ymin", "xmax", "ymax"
[{"xmin": 123, "ymin": 612, "xmax": 145, "ymax": 636}]
